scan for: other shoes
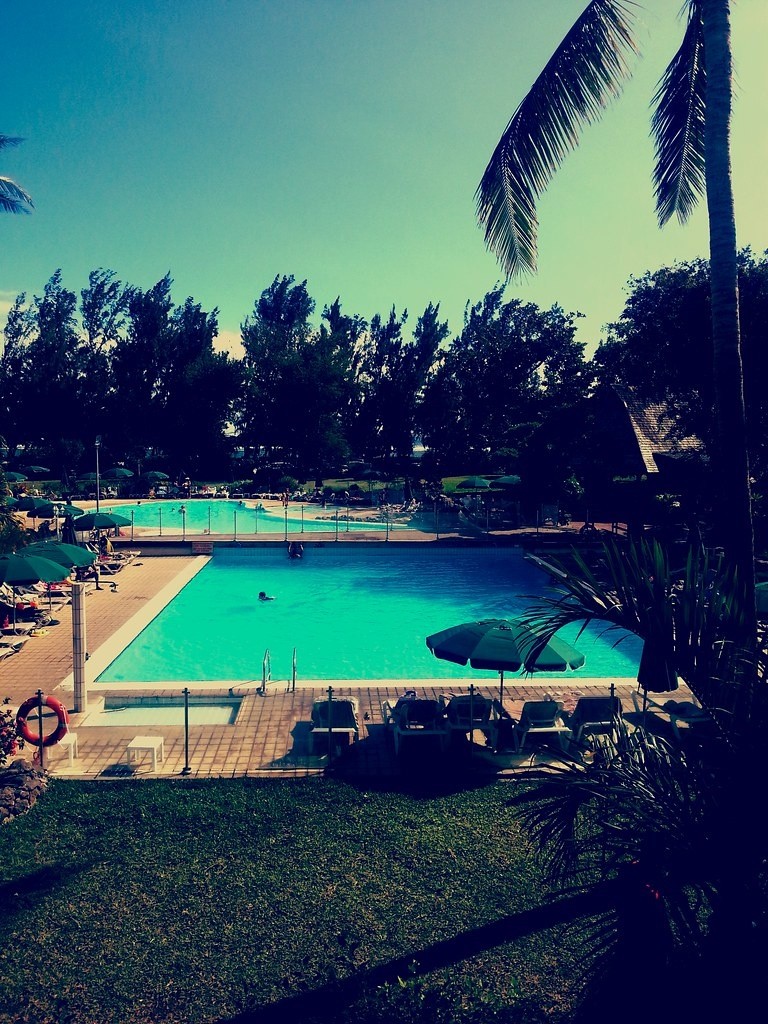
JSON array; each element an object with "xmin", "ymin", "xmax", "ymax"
[{"xmin": 96, "ymin": 586, "xmax": 103, "ymax": 590}]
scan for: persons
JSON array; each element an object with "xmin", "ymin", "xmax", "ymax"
[
  {"xmin": 90, "ymin": 527, "xmax": 100, "ymax": 542},
  {"xmin": 18, "ymin": 485, "xmax": 27, "ymax": 494},
  {"xmin": 280, "ymin": 485, "xmax": 504, "ymax": 522},
  {"xmin": 202, "ymin": 484, "xmax": 208, "ymax": 497},
  {"xmin": 217, "ymin": 485, "xmax": 230, "ymax": 498},
  {"xmin": 173, "ymin": 479, "xmax": 180, "ymax": 499},
  {"xmin": 0, "ymin": 601, "xmax": 44, "ymax": 624},
  {"xmin": 100, "ymin": 535, "xmax": 114, "ymax": 554},
  {"xmin": 148, "ymin": 489, "xmax": 156, "ymax": 498},
  {"xmin": 181, "ymin": 477, "xmax": 192, "ymax": 498},
  {"xmin": 12, "ymin": 485, "xmax": 22, "ymax": 512},
  {"xmin": 74, "ymin": 563, "xmax": 104, "ymax": 590},
  {"xmin": 25, "ymin": 487, "xmax": 31, "ymax": 494},
  {"xmin": 38, "ymin": 520, "xmax": 54, "ymax": 539}
]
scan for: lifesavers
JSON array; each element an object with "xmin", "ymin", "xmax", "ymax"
[{"xmin": 15, "ymin": 697, "xmax": 70, "ymax": 747}]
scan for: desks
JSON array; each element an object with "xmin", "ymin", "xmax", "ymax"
[
  {"xmin": 125, "ymin": 735, "xmax": 164, "ymax": 773},
  {"xmin": 43, "ymin": 732, "xmax": 78, "ymax": 767}
]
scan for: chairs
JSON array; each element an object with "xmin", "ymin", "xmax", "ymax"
[
  {"xmin": 548, "ymin": 690, "xmax": 623, "ymax": 754},
  {"xmin": 632, "ymin": 689, "xmax": 713, "ymax": 742},
  {"xmin": 383, "ymin": 690, "xmax": 447, "ymax": 756},
  {"xmin": 439, "ymin": 692, "xmax": 497, "ymax": 754},
  {"xmin": 0, "ymin": 543, "xmax": 143, "ymax": 660},
  {"xmin": 217, "ymin": 491, "xmax": 369, "ymax": 504},
  {"xmin": 497, "ymin": 697, "xmax": 573, "ymax": 754},
  {"xmin": 309, "ymin": 695, "xmax": 362, "ymax": 752}
]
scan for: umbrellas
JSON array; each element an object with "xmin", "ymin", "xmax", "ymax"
[
  {"xmin": 426, "ymin": 618, "xmax": 585, "ymax": 720},
  {"xmin": 456, "ymin": 474, "xmax": 521, "ymax": 509},
  {"xmin": 0, "ymin": 464, "xmax": 169, "ymax": 632}
]
